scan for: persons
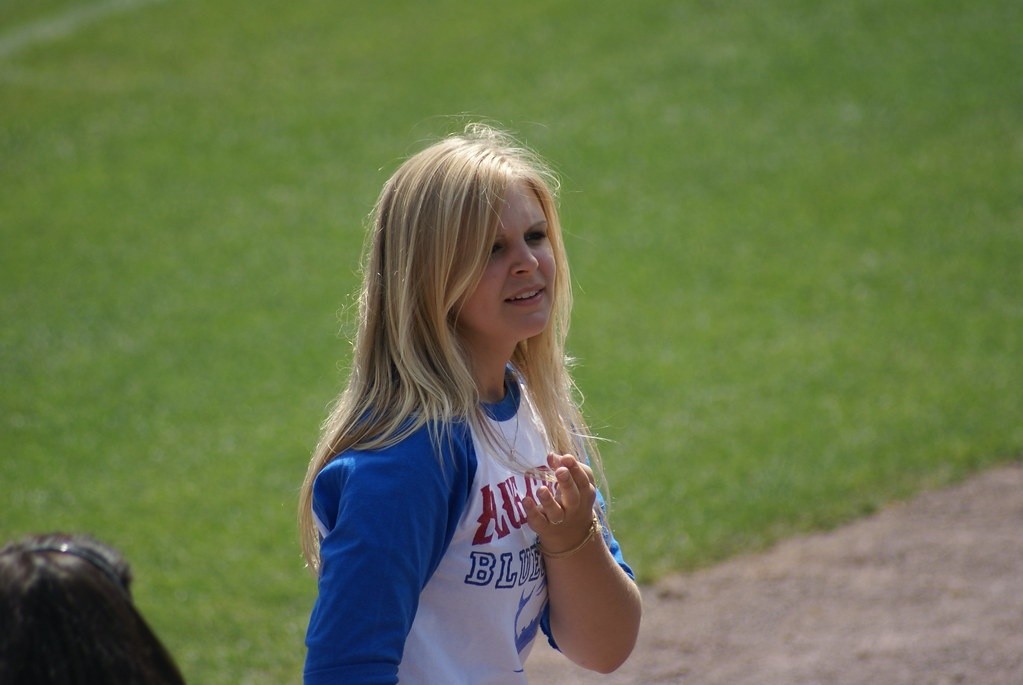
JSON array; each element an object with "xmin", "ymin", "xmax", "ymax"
[
  {"xmin": 1, "ymin": 534, "xmax": 186, "ymax": 685},
  {"xmin": 303, "ymin": 121, "xmax": 641, "ymax": 684}
]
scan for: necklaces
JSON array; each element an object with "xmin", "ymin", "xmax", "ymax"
[{"xmin": 482, "ymin": 379, "xmax": 519, "ymax": 455}]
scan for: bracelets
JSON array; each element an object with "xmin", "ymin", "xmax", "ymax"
[{"xmin": 536, "ymin": 514, "xmax": 597, "ymax": 558}]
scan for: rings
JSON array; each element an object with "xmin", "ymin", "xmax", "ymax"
[{"xmin": 549, "ymin": 520, "xmax": 563, "ymax": 525}]
